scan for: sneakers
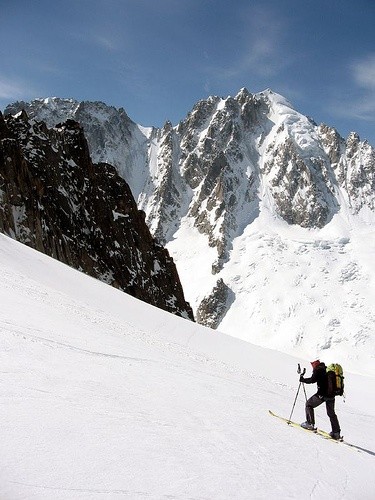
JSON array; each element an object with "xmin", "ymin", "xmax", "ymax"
[
  {"xmin": 301, "ymin": 422, "xmax": 314, "ymax": 430},
  {"xmin": 330, "ymin": 432, "xmax": 340, "ymax": 439}
]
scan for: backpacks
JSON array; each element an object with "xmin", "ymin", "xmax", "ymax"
[{"xmin": 326, "ymin": 364, "xmax": 344, "ymax": 396}]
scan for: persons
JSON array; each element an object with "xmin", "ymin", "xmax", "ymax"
[{"xmin": 299, "ymin": 357, "xmax": 344, "ymax": 441}]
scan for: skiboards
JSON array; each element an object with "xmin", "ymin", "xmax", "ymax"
[{"xmin": 268, "ymin": 409, "xmax": 375, "ymax": 457}]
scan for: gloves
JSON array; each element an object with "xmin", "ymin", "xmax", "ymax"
[{"xmin": 300, "ymin": 377, "xmax": 304, "ymax": 382}]
